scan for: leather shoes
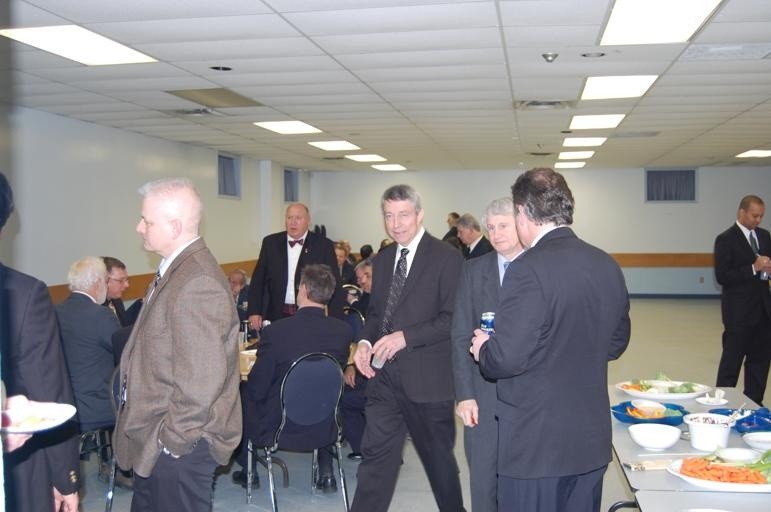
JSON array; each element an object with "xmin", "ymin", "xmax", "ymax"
[
  {"xmin": 102, "ymin": 460, "xmax": 136, "ymax": 492},
  {"xmin": 233, "ymin": 470, "xmax": 259, "ymax": 487},
  {"xmin": 315, "ymin": 473, "xmax": 337, "ymax": 493},
  {"xmin": 346, "ymin": 451, "xmax": 363, "ymax": 461}
]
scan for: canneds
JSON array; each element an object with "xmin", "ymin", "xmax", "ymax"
[
  {"xmin": 240, "ymin": 320, "xmax": 252, "ymax": 343},
  {"xmin": 262, "ymin": 320, "xmax": 272, "ymax": 330},
  {"xmin": 480, "ymin": 312, "xmax": 496, "ymax": 337}
]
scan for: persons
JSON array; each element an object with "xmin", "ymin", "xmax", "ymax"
[
  {"xmin": 351, "ymin": 175, "xmax": 466, "ymax": 512},
  {"xmin": 448, "ymin": 196, "xmax": 531, "ymax": 511},
  {"xmin": 112, "ymin": 177, "xmax": 245, "ymax": 512},
  {"xmin": 470, "ymin": 165, "xmax": 633, "ymax": 511},
  {"xmin": 2, "ymin": 173, "xmax": 82, "ymax": 512},
  {"xmin": 713, "ymin": 194, "xmax": 771, "ymax": 407}
]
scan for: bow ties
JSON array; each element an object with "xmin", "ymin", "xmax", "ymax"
[{"xmin": 288, "ymin": 239, "xmax": 304, "ymax": 248}]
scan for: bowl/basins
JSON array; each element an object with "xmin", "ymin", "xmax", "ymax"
[
  {"xmin": 627, "ymin": 422, "xmax": 682, "ymax": 450},
  {"xmin": 707, "ymin": 407, "xmax": 771, "ymax": 434},
  {"xmin": 683, "ymin": 412, "xmax": 736, "ymax": 453}
]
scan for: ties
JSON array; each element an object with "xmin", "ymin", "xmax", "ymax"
[
  {"xmin": 749, "ymin": 233, "xmax": 758, "ymax": 253},
  {"xmin": 383, "ymin": 248, "xmax": 409, "ymax": 333},
  {"xmin": 503, "ymin": 261, "xmax": 513, "ymax": 272}
]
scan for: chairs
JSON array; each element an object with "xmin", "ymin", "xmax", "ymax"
[
  {"xmin": 341, "ymin": 284, "xmax": 366, "ymax": 345},
  {"xmin": 79, "ymin": 365, "xmax": 123, "ymax": 512},
  {"xmin": 247, "ymin": 351, "xmax": 349, "ymax": 512}
]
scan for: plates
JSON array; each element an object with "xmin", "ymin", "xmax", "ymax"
[
  {"xmin": 695, "ymin": 396, "xmax": 729, "ymax": 407},
  {"xmin": 665, "ymin": 456, "xmax": 770, "ymax": 493},
  {"xmin": 614, "ymin": 378, "xmax": 713, "ymax": 401},
  {"xmin": 714, "ymin": 448, "xmax": 761, "ymax": 464},
  {"xmin": 742, "ymin": 431, "xmax": 771, "ymax": 453},
  {"xmin": 608, "ymin": 401, "xmax": 691, "ymax": 427},
  {"xmin": 1, "ymin": 401, "xmax": 77, "ymax": 434}
]
